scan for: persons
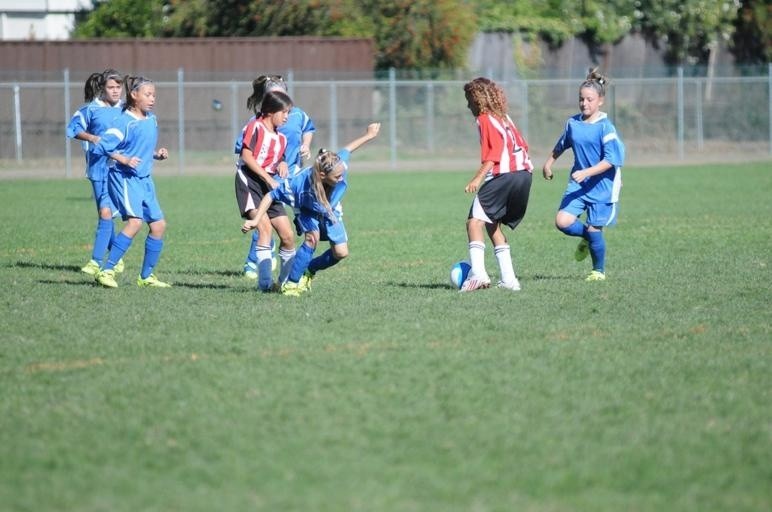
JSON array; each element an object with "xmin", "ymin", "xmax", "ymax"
[
  {"xmin": 459, "ymin": 75, "xmax": 533, "ymax": 294},
  {"xmin": 234, "ymin": 90, "xmax": 297, "ymax": 267},
  {"xmin": 94, "ymin": 73, "xmax": 172, "ymax": 289},
  {"xmin": 66, "ymin": 69, "xmax": 129, "ymax": 276},
  {"xmin": 241, "ymin": 122, "xmax": 377, "ymax": 297},
  {"xmin": 235, "ymin": 74, "xmax": 316, "ymax": 280},
  {"xmin": 542, "ymin": 65, "xmax": 625, "ymax": 283}
]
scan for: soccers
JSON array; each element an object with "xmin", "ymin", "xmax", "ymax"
[{"xmin": 449, "ymin": 262, "xmax": 472, "ymax": 287}]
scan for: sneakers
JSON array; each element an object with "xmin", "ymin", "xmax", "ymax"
[
  {"xmin": 243, "ymin": 258, "xmax": 313, "ymax": 297},
  {"xmin": 574, "ymin": 239, "xmax": 590, "ymax": 262},
  {"xmin": 495, "ymin": 281, "xmax": 522, "ymax": 291},
  {"xmin": 94, "ymin": 269, "xmax": 117, "ymax": 287},
  {"xmin": 459, "ymin": 278, "xmax": 491, "ymax": 295},
  {"xmin": 584, "ymin": 268, "xmax": 608, "ymax": 284},
  {"xmin": 138, "ymin": 273, "xmax": 172, "ymax": 289},
  {"xmin": 112, "ymin": 257, "xmax": 125, "ymax": 273},
  {"xmin": 81, "ymin": 259, "xmax": 98, "ymax": 274}
]
